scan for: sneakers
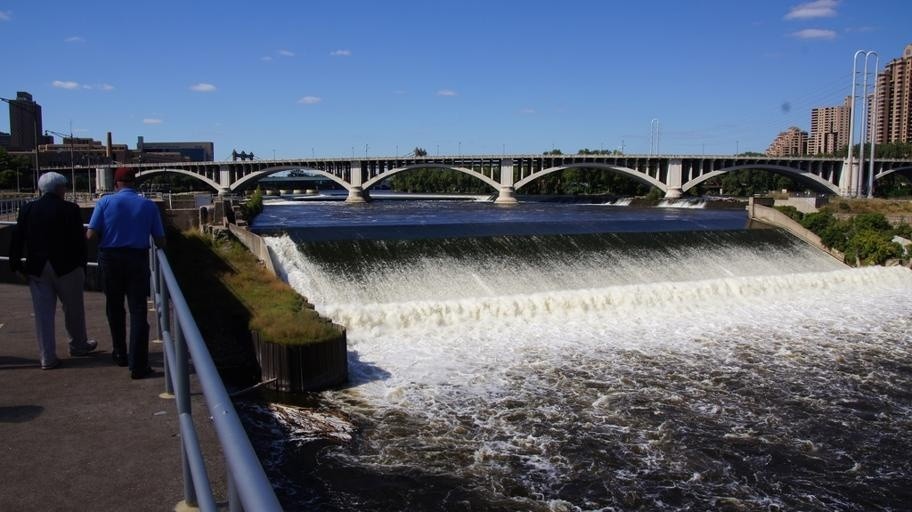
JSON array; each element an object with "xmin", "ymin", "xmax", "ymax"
[
  {"xmin": 131, "ymin": 365, "xmax": 151, "ymax": 379},
  {"xmin": 71, "ymin": 341, "xmax": 97, "ymax": 357},
  {"xmin": 113, "ymin": 352, "xmax": 127, "ymax": 368},
  {"xmin": 42, "ymin": 358, "xmax": 59, "ymax": 369}
]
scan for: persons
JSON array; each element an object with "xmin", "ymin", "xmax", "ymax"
[
  {"xmin": 86, "ymin": 168, "xmax": 167, "ymax": 379},
  {"xmin": 7, "ymin": 171, "xmax": 100, "ymax": 372}
]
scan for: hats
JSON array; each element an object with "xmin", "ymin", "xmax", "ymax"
[{"xmin": 114, "ymin": 167, "xmax": 134, "ymax": 182}]
